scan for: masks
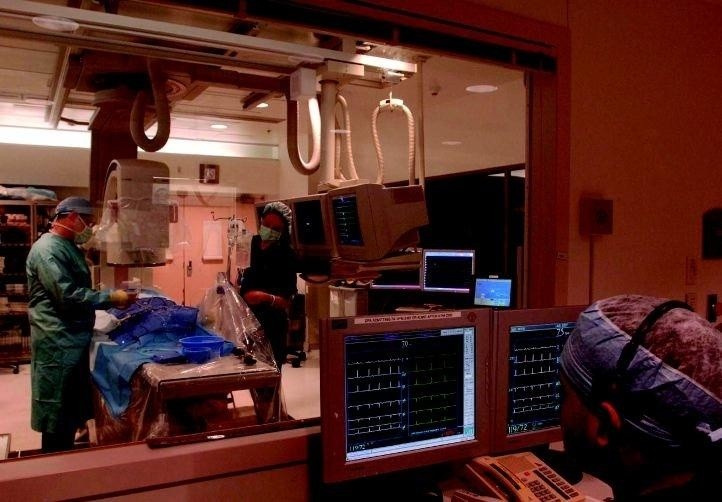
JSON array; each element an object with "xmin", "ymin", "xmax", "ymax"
[
  {"xmin": 258, "ymin": 224, "xmax": 282, "ymax": 242},
  {"xmin": 73, "ymin": 224, "xmax": 94, "ymax": 245}
]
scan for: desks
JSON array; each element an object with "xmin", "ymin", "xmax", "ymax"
[{"xmin": 91, "ymin": 299, "xmax": 281, "ymax": 440}]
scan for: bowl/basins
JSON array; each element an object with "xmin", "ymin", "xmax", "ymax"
[
  {"xmin": 182, "ymin": 346, "xmax": 211, "ymax": 365},
  {"xmin": 179, "ymin": 335, "xmax": 224, "ymax": 358}
]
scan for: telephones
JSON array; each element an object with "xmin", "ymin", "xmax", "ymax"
[{"xmin": 466, "ymin": 451, "xmax": 586, "ymax": 500}]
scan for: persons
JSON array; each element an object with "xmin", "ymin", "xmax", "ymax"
[
  {"xmin": 24, "ymin": 195, "xmax": 139, "ymax": 451},
  {"xmin": 215, "ymin": 201, "xmax": 299, "ymax": 427},
  {"xmin": 555, "ymin": 293, "xmax": 722, "ymax": 502}
]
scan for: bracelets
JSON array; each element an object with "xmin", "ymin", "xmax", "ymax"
[{"xmin": 269, "ymin": 294, "xmax": 276, "ymax": 306}]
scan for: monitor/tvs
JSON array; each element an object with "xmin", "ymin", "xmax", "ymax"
[
  {"xmin": 254, "ymin": 199, "xmax": 296, "ymax": 250},
  {"xmin": 290, "ymin": 192, "xmax": 339, "ymax": 258},
  {"xmin": 327, "ymin": 182, "xmax": 430, "ymax": 261},
  {"xmin": 492, "ymin": 304, "xmax": 592, "ymax": 484},
  {"xmin": 423, "ymin": 248, "xmax": 476, "ymax": 294},
  {"xmin": 318, "ymin": 307, "xmax": 494, "ymax": 486},
  {"xmin": 469, "ymin": 274, "xmax": 517, "ymax": 310}
]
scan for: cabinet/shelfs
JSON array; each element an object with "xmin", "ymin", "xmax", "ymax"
[{"xmin": 0, "ymin": 199, "xmax": 62, "ymax": 367}]
